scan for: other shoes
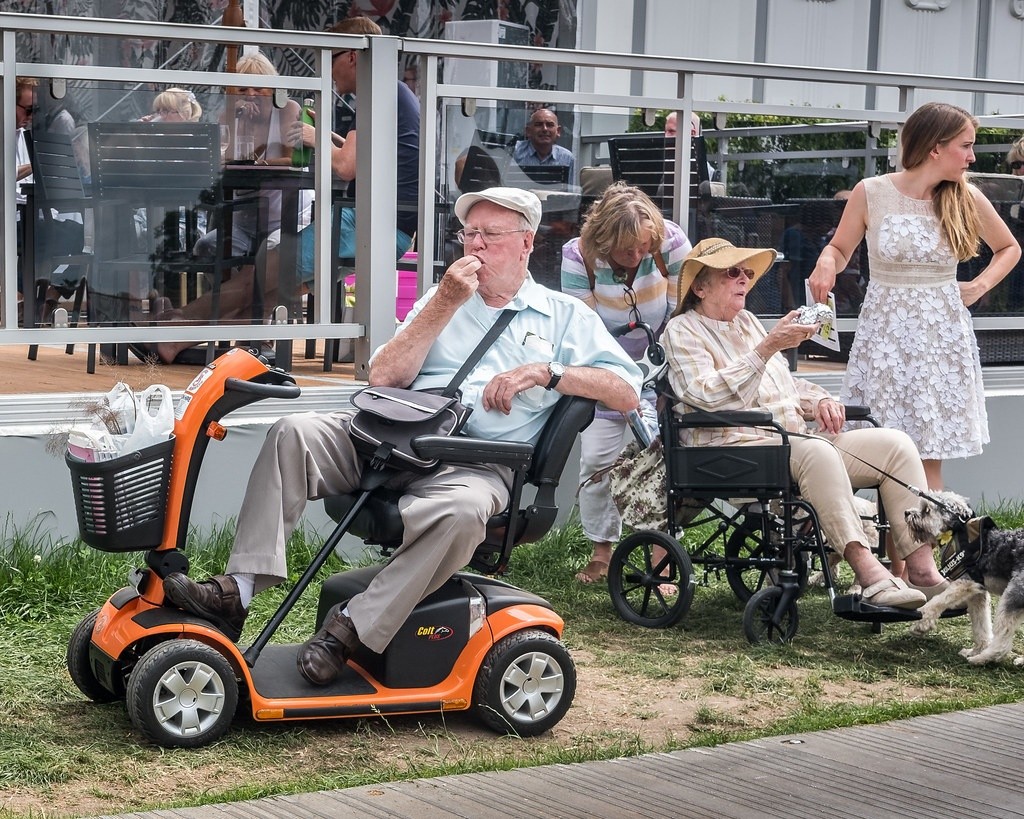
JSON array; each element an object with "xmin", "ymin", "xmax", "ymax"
[
  {"xmin": 17, "ymin": 300, "xmax": 24, "ymax": 322},
  {"xmin": 906, "ymin": 576, "xmax": 969, "ymax": 610},
  {"xmin": 40, "ymin": 298, "xmax": 61, "ymax": 325},
  {"xmin": 861, "ymin": 577, "xmax": 927, "ymax": 609},
  {"xmin": 261, "ymin": 342, "xmax": 276, "ymax": 364},
  {"xmin": 575, "ymin": 561, "xmax": 608, "ymax": 584}
]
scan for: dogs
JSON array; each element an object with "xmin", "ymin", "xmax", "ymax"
[{"xmin": 905, "ymin": 488, "xmax": 1024, "ymax": 667}]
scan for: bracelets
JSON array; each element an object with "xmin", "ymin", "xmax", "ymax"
[{"xmin": 753, "ymin": 349, "xmax": 767, "ymax": 364}]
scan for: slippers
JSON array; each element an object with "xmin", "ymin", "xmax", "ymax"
[{"xmin": 127, "ymin": 320, "xmax": 165, "ymax": 366}]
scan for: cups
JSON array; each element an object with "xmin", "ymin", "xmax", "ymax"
[
  {"xmin": 234, "ymin": 136, "xmax": 255, "ymax": 160},
  {"xmin": 149, "ymin": 115, "xmax": 163, "ymax": 122}
]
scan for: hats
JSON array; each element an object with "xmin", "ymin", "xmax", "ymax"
[
  {"xmin": 454, "ymin": 187, "xmax": 542, "ymax": 236},
  {"xmin": 673, "ymin": 236, "xmax": 777, "ymax": 318}
]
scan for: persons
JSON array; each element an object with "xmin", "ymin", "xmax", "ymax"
[
  {"xmin": 560, "ymin": 181, "xmax": 693, "ymax": 596},
  {"xmin": 664, "ymin": 238, "xmax": 970, "ymax": 607},
  {"xmin": 810, "ymin": 103, "xmax": 1021, "ymax": 579},
  {"xmin": 1001, "ymin": 134, "xmax": 1024, "ymax": 249},
  {"xmin": 504, "ymin": 108, "xmax": 582, "ymax": 272},
  {"xmin": 777, "ymin": 200, "xmax": 862, "ymax": 340},
  {"xmin": 822, "ymin": 188, "xmax": 869, "ymax": 315},
  {"xmin": 656, "ymin": 111, "xmax": 714, "ymax": 196},
  {"xmin": 17, "ymin": 15, "xmax": 425, "ymax": 364},
  {"xmin": 161, "ymin": 188, "xmax": 643, "ymax": 686}
]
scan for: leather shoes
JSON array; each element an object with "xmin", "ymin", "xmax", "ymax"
[
  {"xmin": 297, "ymin": 599, "xmax": 362, "ymax": 688},
  {"xmin": 162, "ymin": 571, "xmax": 250, "ymax": 643}
]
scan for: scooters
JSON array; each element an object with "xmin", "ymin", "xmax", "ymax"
[{"xmin": 67, "ymin": 346, "xmax": 595, "ymax": 747}]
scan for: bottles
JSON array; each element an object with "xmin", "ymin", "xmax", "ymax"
[{"xmin": 292, "ymin": 98, "xmax": 315, "ymax": 167}]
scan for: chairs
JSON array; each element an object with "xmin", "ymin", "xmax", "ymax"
[{"xmin": 322, "ymin": 394, "xmax": 597, "ymax": 549}]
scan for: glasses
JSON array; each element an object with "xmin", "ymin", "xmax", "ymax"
[
  {"xmin": 717, "ymin": 266, "xmax": 755, "ymax": 281},
  {"xmin": 153, "ymin": 110, "xmax": 179, "ymax": 119},
  {"xmin": 1009, "ymin": 159, "xmax": 1024, "ymax": 169},
  {"xmin": 17, "ymin": 103, "xmax": 40, "ymax": 116},
  {"xmin": 457, "ymin": 227, "xmax": 527, "ymax": 244},
  {"xmin": 623, "ymin": 286, "xmax": 642, "ymax": 323}
]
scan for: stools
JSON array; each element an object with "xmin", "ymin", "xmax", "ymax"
[{"xmin": 22, "ymin": 117, "xmax": 1024, "ymax": 382}]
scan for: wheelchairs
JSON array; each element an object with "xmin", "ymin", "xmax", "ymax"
[{"xmin": 606, "ymin": 320, "xmax": 969, "ymax": 645}]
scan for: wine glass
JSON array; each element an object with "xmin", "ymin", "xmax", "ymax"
[{"xmin": 221, "ymin": 125, "xmax": 230, "ymax": 165}]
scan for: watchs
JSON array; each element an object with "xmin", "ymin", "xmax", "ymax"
[{"xmin": 544, "ymin": 361, "xmax": 566, "ymax": 392}]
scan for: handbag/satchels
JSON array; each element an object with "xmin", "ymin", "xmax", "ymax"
[
  {"xmin": 69, "ymin": 381, "xmax": 174, "ymax": 459},
  {"xmin": 609, "ymin": 440, "xmax": 716, "ymax": 529},
  {"xmin": 348, "ymin": 382, "xmax": 473, "ymax": 476}
]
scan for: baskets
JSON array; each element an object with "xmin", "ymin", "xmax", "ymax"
[{"xmin": 66, "ymin": 434, "xmax": 176, "ymax": 553}]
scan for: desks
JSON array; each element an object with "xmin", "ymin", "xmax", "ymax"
[{"xmin": 164, "ymin": 164, "xmax": 348, "ymax": 372}]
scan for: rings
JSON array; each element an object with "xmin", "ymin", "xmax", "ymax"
[{"xmin": 805, "ymin": 333, "xmax": 810, "ymax": 339}]
